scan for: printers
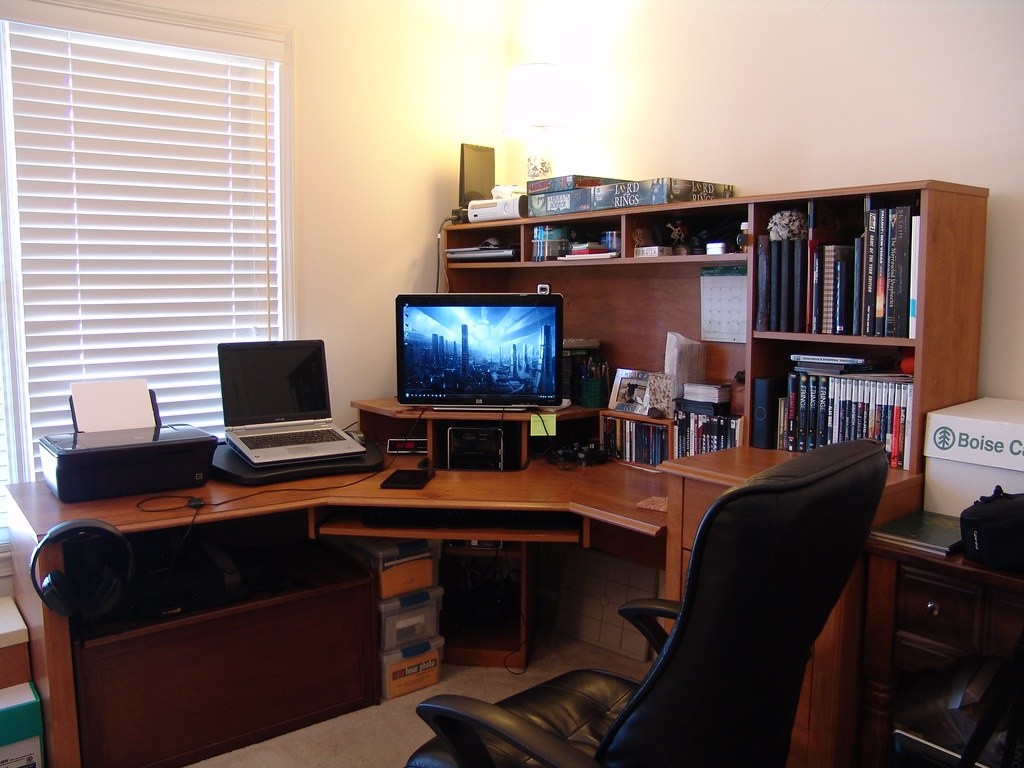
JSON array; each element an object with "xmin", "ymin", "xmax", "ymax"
[{"xmin": 38, "ymin": 390, "xmax": 218, "ymax": 504}]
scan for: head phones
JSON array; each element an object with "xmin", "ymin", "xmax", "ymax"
[{"xmin": 28, "ymin": 518, "xmax": 133, "ymax": 614}]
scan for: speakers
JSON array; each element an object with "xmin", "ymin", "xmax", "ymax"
[{"xmin": 459, "ymin": 143, "xmax": 496, "ymax": 208}]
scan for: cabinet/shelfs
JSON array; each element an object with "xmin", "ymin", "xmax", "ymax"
[
  {"xmin": 439, "ymin": 196, "xmax": 748, "ymax": 452},
  {"xmin": 659, "ymin": 444, "xmax": 924, "ymax": 767},
  {"xmin": 749, "ymin": 178, "xmax": 991, "ymax": 472},
  {"xmin": 869, "ymin": 510, "xmax": 1024, "ymax": 768}
]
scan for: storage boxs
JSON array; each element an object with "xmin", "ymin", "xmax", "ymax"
[
  {"xmin": 525, "ymin": 174, "xmax": 735, "ymax": 218},
  {"xmin": 0, "ymin": 681, "xmax": 45, "ymax": 768},
  {"xmin": 373, "ymin": 547, "xmax": 437, "ymax": 599},
  {"xmin": 0, "ymin": 590, "xmax": 36, "ymax": 691},
  {"xmin": 377, "ymin": 635, "xmax": 447, "ymax": 698},
  {"xmin": 924, "ymin": 397, "xmax": 1023, "ymax": 518},
  {"xmin": 380, "ymin": 587, "xmax": 443, "ymax": 652}
]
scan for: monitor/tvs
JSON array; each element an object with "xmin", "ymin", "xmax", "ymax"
[{"xmin": 395, "ymin": 293, "xmax": 563, "ymax": 411}]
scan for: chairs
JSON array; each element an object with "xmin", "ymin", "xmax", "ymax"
[{"xmin": 407, "ymin": 437, "xmax": 892, "ymax": 768}]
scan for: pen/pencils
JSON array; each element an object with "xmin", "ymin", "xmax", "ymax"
[{"xmin": 580, "ymin": 357, "xmax": 611, "ymax": 399}]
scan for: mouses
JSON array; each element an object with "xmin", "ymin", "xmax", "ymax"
[{"xmin": 480, "ymin": 237, "xmax": 502, "ymax": 248}]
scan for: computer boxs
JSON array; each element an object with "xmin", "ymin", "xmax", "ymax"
[{"xmin": 445, "ymin": 423, "xmax": 508, "ymax": 549}]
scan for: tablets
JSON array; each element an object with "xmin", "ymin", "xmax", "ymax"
[{"xmin": 380, "ymin": 469, "xmax": 436, "ymax": 489}]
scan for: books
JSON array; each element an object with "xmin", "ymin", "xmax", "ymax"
[
  {"xmin": 753, "ymin": 201, "xmax": 917, "ymax": 341},
  {"xmin": 869, "ymin": 508, "xmax": 970, "ymax": 559},
  {"xmin": 599, "ymin": 383, "xmax": 747, "ymax": 472},
  {"xmin": 776, "ymin": 352, "xmax": 912, "ymax": 472}
]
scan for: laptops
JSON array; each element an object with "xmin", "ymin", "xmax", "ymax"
[{"xmin": 217, "ymin": 339, "xmax": 366, "ymax": 468}]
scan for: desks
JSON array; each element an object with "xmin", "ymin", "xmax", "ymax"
[{"xmin": 6, "ymin": 394, "xmax": 666, "ymax": 768}]
